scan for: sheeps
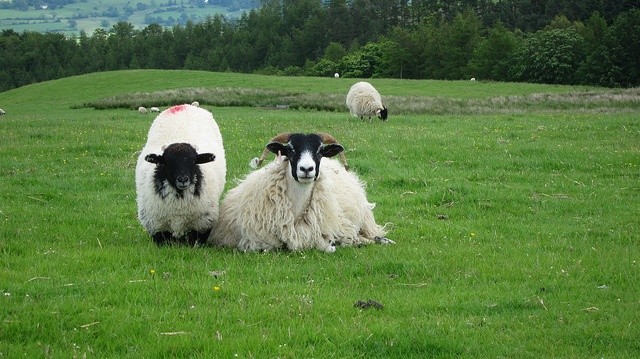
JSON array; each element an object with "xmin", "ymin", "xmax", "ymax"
[
  {"xmin": 346, "ymin": 81, "xmax": 387, "ymax": 122},
  {"xmin": 207, "ymin": 132, "xmax": 397, "ymax": 254},
  {"xmin": 134, "ymin": 104, "xmax": 227, "ymax": 249}
]
